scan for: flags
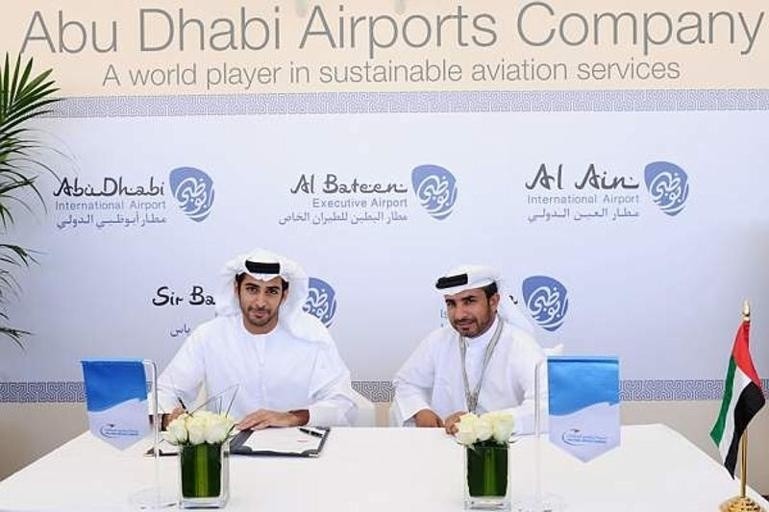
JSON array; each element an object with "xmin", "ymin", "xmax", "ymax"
[{"xmin": 709, "ymin": 299, "xmax": 766, "ymax": 479}]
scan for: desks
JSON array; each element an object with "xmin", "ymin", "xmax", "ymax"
[{"xmin": 0, "ymin": 421, "xmax": 768, "ymax": 512}]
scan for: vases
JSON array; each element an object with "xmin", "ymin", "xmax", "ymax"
[
  {"xmin": 176, "ymin": 439, "xmax": 230, "ymax": 508},
  {"xmin": 463, "ymin": 435, "xmax": 508, "ymax": 510}
]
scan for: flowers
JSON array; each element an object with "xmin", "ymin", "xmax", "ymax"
[
  {"xmin": 159, "ymin": 383, "xmax": 239, "ymax": 446},
  {"xmin": 454, "ymin": 413, "xmax": 514, "ymax": 446}
]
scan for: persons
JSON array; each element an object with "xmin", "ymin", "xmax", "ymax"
[
  {"xmin": 148, "ymin": 253, "xmax": 357, "ymax": 431},
  {"xmin": 391, "ymin": 265, "xmax": 549, "ymax": 437}
]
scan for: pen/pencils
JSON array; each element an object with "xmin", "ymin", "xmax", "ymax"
[
  {"xmin": 177, "ymin": 396, "xmax": 189, "ymax": 414},
  {"xmin": 297, "ymin": 426, "xmax": 324, "ymax": 438}
]
